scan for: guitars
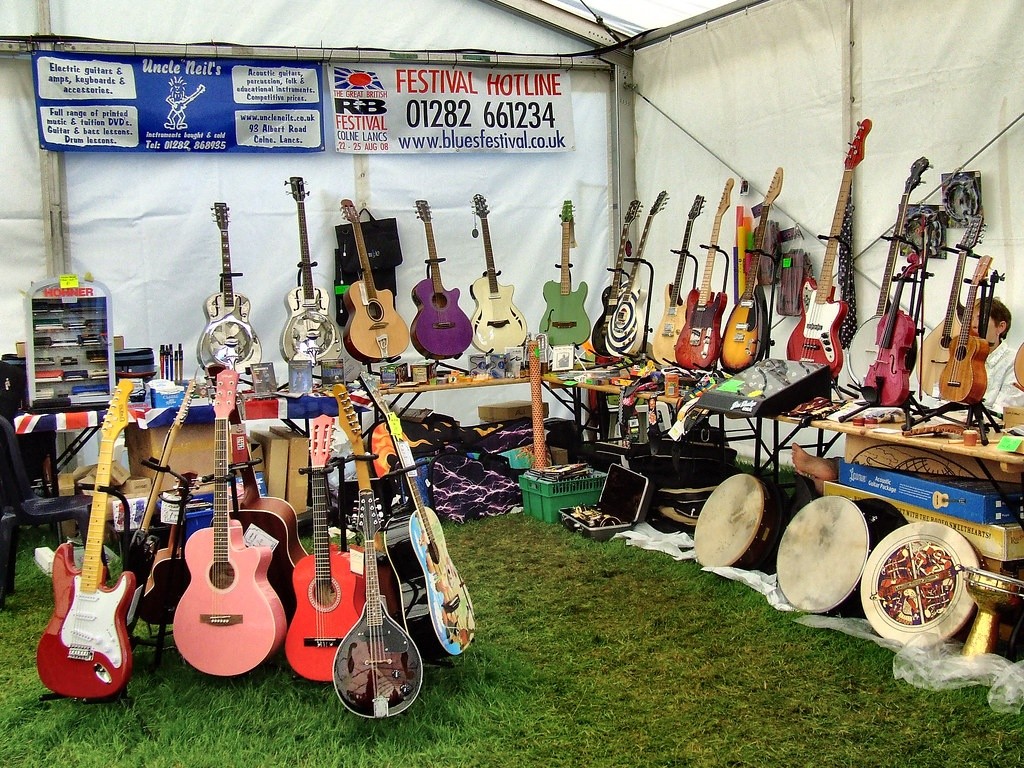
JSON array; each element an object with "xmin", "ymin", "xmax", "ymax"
[
  {"xmin": 539, "ymin": 118, "xmax": 993, "ymax": 408},
  {"xmin": 1014, "ymin": 342, "xmax": 1024, "ymax": 388},
  {"xmin": 37, "ymin": 356, "xmax": 480, "ymax": 719},
  {"xmin": 197, "ymin": 202, "xmax": 265, "ymax": 381},
  {"xmin": 279, "ymin": 175, "xmax": 343, "ymax": 372},
  {"xmin": 410, "ymin": 192, "xmax": 526, "ymax": 360},
  {"xmin": 336, "ymin": 197, "xmax": 411, "ymax": 366}
]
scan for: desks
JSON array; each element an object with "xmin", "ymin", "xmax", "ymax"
[
  {"xmin": 14, "ymin": 390, "xmax": 372, "ymax": 582},
  {"xmin": 281, "ymin": 363, "xmax": 593, "ymax": 464},
  {"xmin": 540, "ymin": 366, "xmax": 1024, "ymax": 660}
]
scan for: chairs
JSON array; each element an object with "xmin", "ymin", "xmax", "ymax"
[{"xmin": 0, "ymin": 415, "xmax": 110, "ymax": 610}]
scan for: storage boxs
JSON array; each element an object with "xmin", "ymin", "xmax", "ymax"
[
  {"xmin": 74, "ymin": 459, "xmax": 130, "ymax": 486},
  {"xmin": 106, "ymin": 513, "xmax": 178, "ymax": 589},
  {"xmin": 155, "ymin": 492, "xmax": 216, "ymax": 544},
  {"xmin": 380, "ymin": 363, "xmax": 408, "ymax": 384},
  {"xmin": 76, "ymin": 475, "xmax": 95, "ymax": 495},
  {"xmin": 250, "ymin": 429, "xmax": 290, "ymax": 502},
  {"xmin": 478, "ymin": 400, "xmax": 549, "ymax": 424},
  {"xmin": 123, "ymin": 475, "xmax": 152, "ymax": 495},
  {"xmin": 73, "ymin": 467, "xmax": 97, "ymax": 481},
  {"xmin": 150, "ymin": 386, "xmax": 185, "ymax": 408},
  {"xmin": 58, "ymin": 473, "xmax": 76, "ymax": 541},
  {"xmin": 410, "ymin": 361, "xmax": 437, "ymax": 384},
  {"xmin": 119, "ymin": 379, "xmax": 153, "ymax": 408},
  {"xmin": 124, "ymin": 420, "xmax": 233, "ymax": 494},
  {"xmin": 519, "ymin": 469, "xmax": 608, "ymax": 524},
  {"xmin": 551, "ymin": 344, "xmax": 576, "ymax": 371},
  {"xmin": 822, "ymin": 481, "xmax": 1024, "ymax": 561},
  {"xmin": 269, "ymin": 426, "xmax": 309, "ymax": 515},
  {"xmin": 469, "ymin": 353, "xmax": 510, "ymax": 379},
  {"xmin": 839, "ymin": 460, "xmax": 1024, "ymax": 524}
]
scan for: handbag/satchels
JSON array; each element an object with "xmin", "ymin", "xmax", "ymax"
[
  {"xmin": 776, "ymin": 224, "xmax": 814, "ymax": 317},
  {"xmin": 334, "ymin": 207, "xmax": 403, "ymax": 327},
  {"xmin": 753, "ymin": 220, "xmax": 783, "ymax": 285}
]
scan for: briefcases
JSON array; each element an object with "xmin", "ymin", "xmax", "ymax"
[{"xmin": 557, "ymin": 462, "xmax": 652, "ymax": 541}]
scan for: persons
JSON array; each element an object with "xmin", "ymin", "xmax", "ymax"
[{"xmin": 791, "ymin": 297, "xmax": 1024, "ymax": 494}]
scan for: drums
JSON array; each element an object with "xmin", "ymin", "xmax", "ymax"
[
  {"xmin": 860, "ymin": 521, "xmax": 991, "ymax": 646},
  {"xmin": 776, "ymin": 495, "xmax": 879, "ymax": 616},
  {"xmin": 694, "ymin": 473, "xmax": 783, "ymax": 570}
]
